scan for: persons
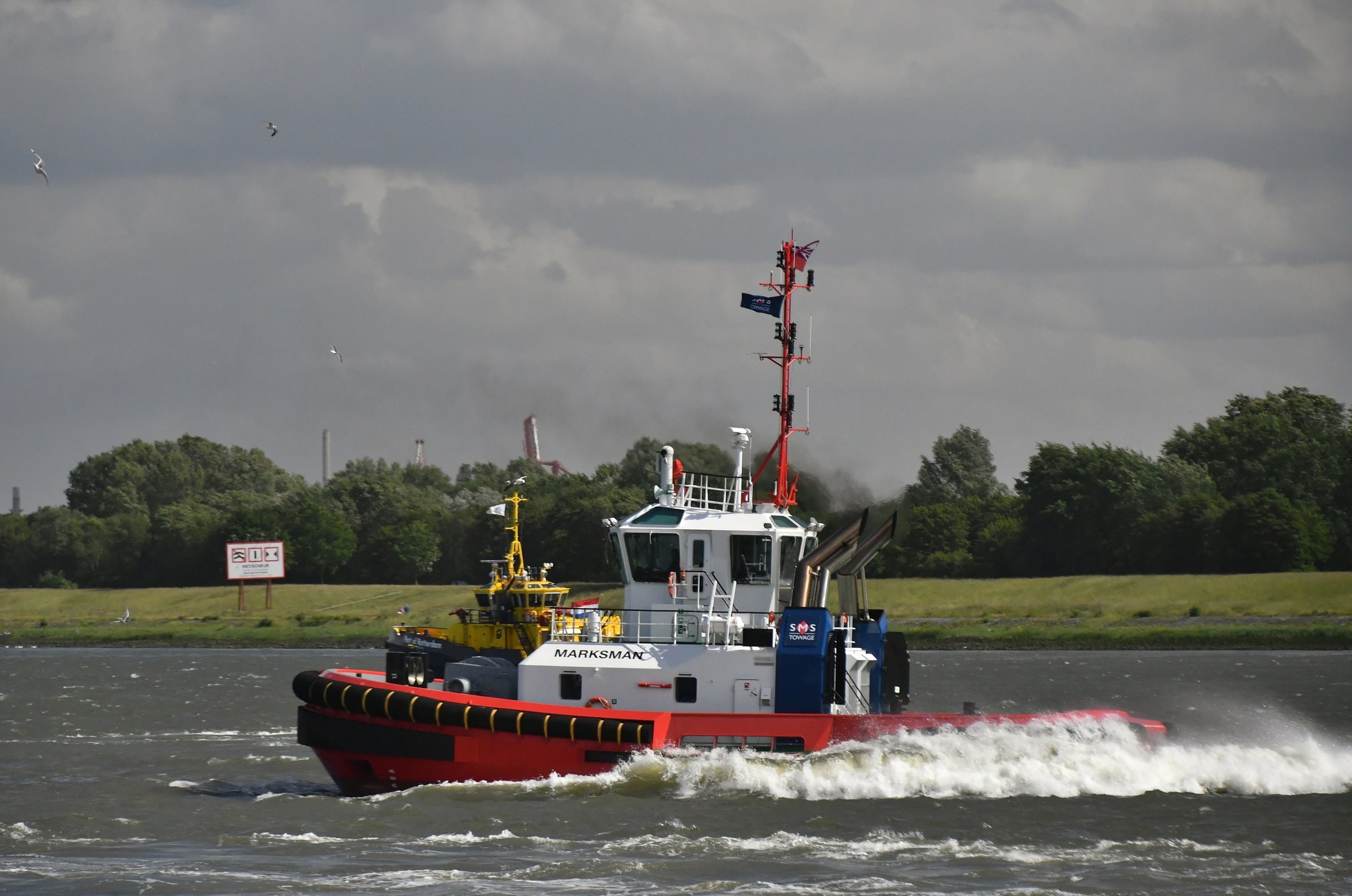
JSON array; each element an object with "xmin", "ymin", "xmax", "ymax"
[
  {"xmin": 405, "ymin": 604, "xmax": 410, "ymax": 613},
  {"xmin": 397, "ymin": 606, "xmax": 405, "ymax": 614}
]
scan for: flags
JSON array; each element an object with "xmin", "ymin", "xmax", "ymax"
[
  {"xmin": 791, "ymin": 245, "xmax": 815, "ymax": 261},
  {"xmin": 740, "ymin": 292, "xmax": 786, "ymax": 318},
  {"xmin": 569, "ymin": 598, "xmax": 599, "ymax": 619},
  {"xmin": 486, "ymin": 503, "xmax": 506, "ymax": 516}
]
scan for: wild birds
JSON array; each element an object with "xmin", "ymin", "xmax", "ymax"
[
  {"xmin": 30, "ymin": 148, "xmax": 50, "ymax": 188},
  {"xmin": 111, "ymin": 606, "xmax": 130, "ymax": 623},
  {"xmin": 329, "ymin": 344, "xmax": 344, "ymax": 364},
  {"xmin": 264, "ymin": 121, "xmax": 279, "ymax": 137}
]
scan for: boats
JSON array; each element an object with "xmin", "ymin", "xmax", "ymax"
[{"xmin": 293, "ymin": 230, "xmax": 1173, "ymax": 793}]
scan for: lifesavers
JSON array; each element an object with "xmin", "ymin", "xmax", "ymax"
[
  {"xmin": 585, "ymin": 697, "xmax": 611, "ymax": 709},
  {"xmin": 841, "ymin": 612, "xmax": 848, "ymax": 625},
  {"xmin": 769, "ymin": 611, "xmax": 782, "ymax": 636},
  {"xmin": 668, "ymin": 571, "xmax": 678, "ymax": 599}
]
